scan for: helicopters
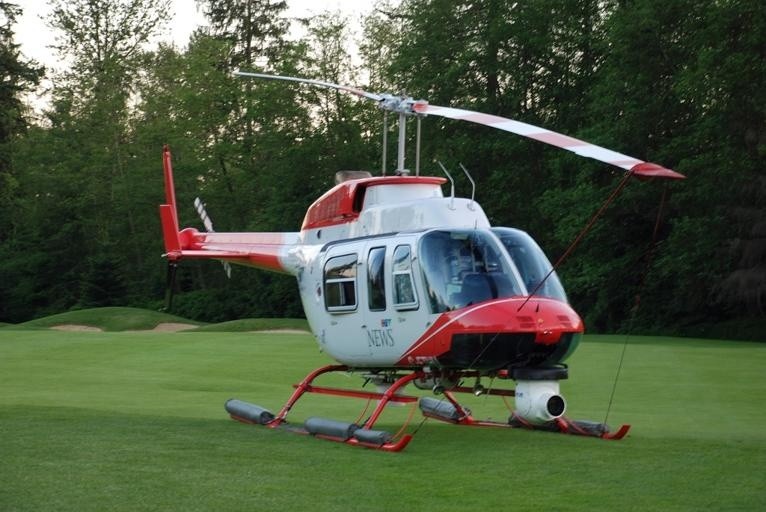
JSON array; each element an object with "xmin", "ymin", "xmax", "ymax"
[{"xmin": 153, "ymin": 64, "xmax": 686, "ymax": 458}]
